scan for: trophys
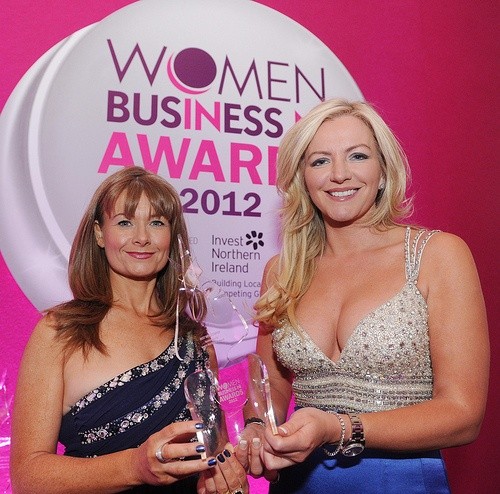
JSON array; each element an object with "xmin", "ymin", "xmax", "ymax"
[{"xmin": 164, "ymin": 233, "xmax": 280, "ymax": 473}]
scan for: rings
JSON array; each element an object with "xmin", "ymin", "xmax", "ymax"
[
  {"xmin": 156, "ymin": 446, "xmax": 166, "ymax": 462},
  {"xmin": 232, "ymin": 488, "xmax": 242, "ymax": 494}
]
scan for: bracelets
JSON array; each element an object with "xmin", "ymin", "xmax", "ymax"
[
  {"xmin": 244, "ymin": 417, "xmax": 266, "ymax": 427},
  {"xmin": 321, "ymin": 412, "xmax": 344, "ymax": 457}
]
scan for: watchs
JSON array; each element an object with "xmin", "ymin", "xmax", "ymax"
[{"xmin": 336, "ymin": 408, "xmax": 365, "ymax": 457}]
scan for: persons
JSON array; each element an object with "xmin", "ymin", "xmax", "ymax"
[
  {"xmin": 11, "ymin": 166, "xmax": 251, "ymax": 494},
  {"xmin": 238, "ymin": 99, "xmax": 490, "ymax": 494}
]
